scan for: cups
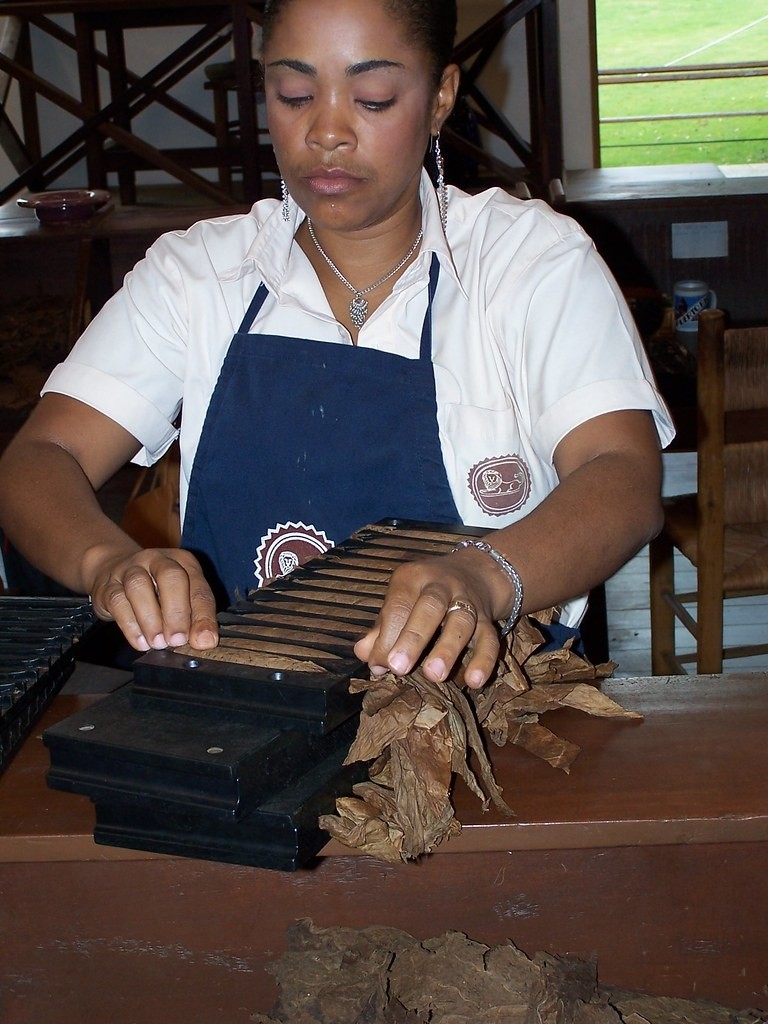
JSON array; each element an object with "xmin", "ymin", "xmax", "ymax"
[{"xmin": 673, "ymin": 280, "xmax": 717, "ymax": 332}]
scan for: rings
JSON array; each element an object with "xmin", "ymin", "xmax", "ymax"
[{"xmin": 448, "ymin": 599, "xmax": 476, "ymax": 617}]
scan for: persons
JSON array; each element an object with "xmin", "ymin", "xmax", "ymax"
[{"xmin": 0, "ymin": 0, "xmax": 676, "ymax": 690}]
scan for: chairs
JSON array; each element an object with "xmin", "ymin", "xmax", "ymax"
[
  {"xmin": 648, "ymin": 310, "xmax": 768, "ymax": 679},
  {"xmin": 0, "ymin": 238, "xmax": 98, "ymax": 464}
]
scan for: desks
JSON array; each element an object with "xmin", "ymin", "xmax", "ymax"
[{"xmin": 1, "ymin": 161, "xmax": 767, "ymax": 672}]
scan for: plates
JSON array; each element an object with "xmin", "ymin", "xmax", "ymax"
[{"xmin": 18, "ymin": 189, "xmax": 109, "ymax": 224}]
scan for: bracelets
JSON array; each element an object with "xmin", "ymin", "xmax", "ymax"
[{"xmin": 451, "ymin": 539, "xmax": 522, "ymax": 637}]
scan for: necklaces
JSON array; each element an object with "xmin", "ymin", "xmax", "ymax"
[{"xmin": 308, "ymin": 216, "xmax": 423, "ymax": 329}]
